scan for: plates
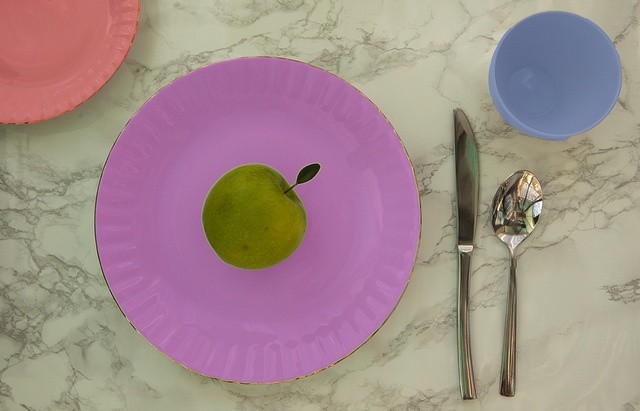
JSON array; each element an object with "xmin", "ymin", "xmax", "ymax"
[
  {"xmin": 0, "ymin": 0, "xmax": 140, "ymax": 127},
  {"xmin": 92, "ymin": 54, "xmax": 425, "ymax": 387}
]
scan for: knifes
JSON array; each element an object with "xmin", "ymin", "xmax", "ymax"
[{"xmin": 452, "ymin": 106, "xmax": 482, "ymax": 400}]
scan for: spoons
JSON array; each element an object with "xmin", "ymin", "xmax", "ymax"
[{"xmin": 491, "ymin": 169, "xmax": 545, "ymax": 397}]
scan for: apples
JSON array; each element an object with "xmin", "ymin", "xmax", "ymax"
[{"xmin": 202, "ymin": 163, "xmax": 321, "ymax": 269}]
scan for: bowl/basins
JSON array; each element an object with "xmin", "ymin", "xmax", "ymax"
[{"xmin": 486, "ymin": 9, "xmax": 624, "ymax": 143}]
